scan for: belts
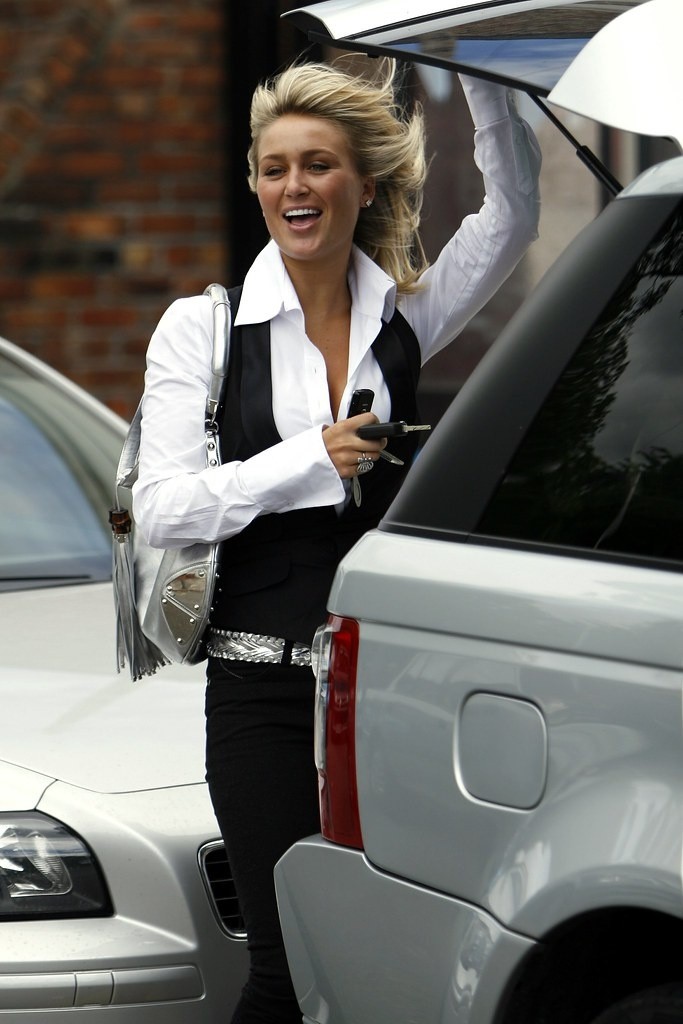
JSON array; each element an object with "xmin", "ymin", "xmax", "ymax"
[{"xmin": 200, "ymin": 628, "xmax": 310, "ymax": 668}]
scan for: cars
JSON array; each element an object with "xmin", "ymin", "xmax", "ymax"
[{"xmin": 1, "ymin": 331, "xmax": 254, "ymax": 1022}]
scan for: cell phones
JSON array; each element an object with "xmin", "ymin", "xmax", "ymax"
[{"xmin": 347, "ymin": 389, "xmax": 374, "ymax": 420}]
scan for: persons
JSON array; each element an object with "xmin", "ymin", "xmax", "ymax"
[{"xmin": 132, "ymin": 65, "xmax": 543, "ymax": 1024}]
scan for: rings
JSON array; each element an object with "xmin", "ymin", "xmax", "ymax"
[{"xmin": 357, "ymin": 453, "xmax": 373, "ymax": 472}]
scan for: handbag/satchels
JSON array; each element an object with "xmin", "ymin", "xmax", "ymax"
[{"xmin": 99, "ymin": 280, "xmax": 237, "ymax": 682}]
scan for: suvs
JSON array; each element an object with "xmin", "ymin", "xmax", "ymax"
[{"xmin": 270, "ymin": 3, "xmax": 683, "ymax": 1022}]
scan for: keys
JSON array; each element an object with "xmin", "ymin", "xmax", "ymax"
[{"xmin": 347, "ymin": 389, "xmax": 430, "ymax": 464}]
